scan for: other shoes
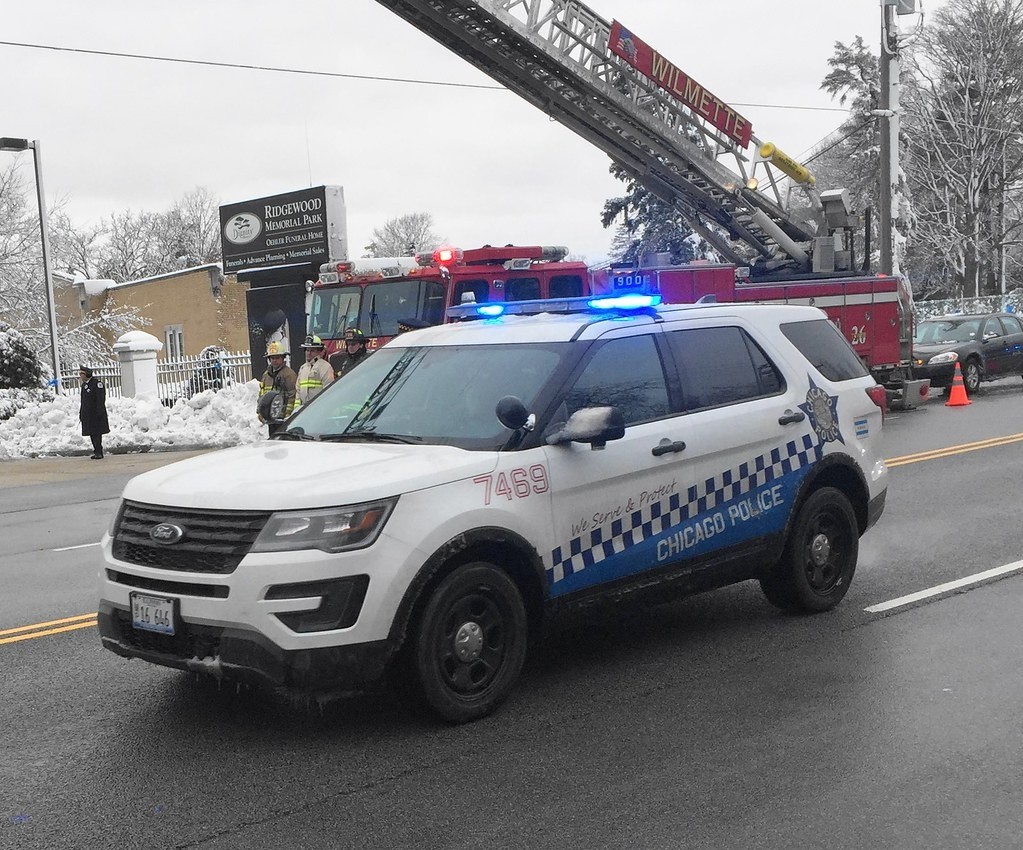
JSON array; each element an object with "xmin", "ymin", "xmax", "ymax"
[
  {"xmin": 95, "ymin": 454, "xmax": 102, "ymax": 459},
  {"xmin": 91, "ymin": 455, "xmax": 95, "ymax": 459}
]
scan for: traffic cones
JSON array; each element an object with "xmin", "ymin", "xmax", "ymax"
[{"xmin": 944, "ymin": 361, "xmax": 974, "ymax": 407}]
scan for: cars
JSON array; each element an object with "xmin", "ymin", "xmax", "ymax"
[{"xmin": 913, "ymin": 313, "xmax": 1022, "ymax": 395}]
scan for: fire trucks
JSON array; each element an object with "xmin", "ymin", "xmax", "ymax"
[{"xmin": 286, "ymin": 1, "xmax": 916, "ymax": 406}]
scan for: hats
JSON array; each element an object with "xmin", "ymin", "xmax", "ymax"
[{"xmin": 79, "ymin": 366, "xmax": 93, "ymax": 378}]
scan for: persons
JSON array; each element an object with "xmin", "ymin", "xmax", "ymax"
[
  {"xmin": 294, "ymin": 335, "xmax": 335, "ymax": 410},
  {"xmin": 79, "ymin": 366, "xmax": 110, "ymax": 459},
  {"xmin": 334, "ymin": 328, "xmax": 368, "ymax": 377},
  {"xmin": 257, "ymin": 342, "xmax": 297, "ymax": 435}
]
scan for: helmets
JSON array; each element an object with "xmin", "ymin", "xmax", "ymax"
[
  {"xmin": 263, "ymin": 343, "xmax": 290, "ymax": 358},
  {"xmin": 345, "ymin": 327, "xmax": 370, "ymax": 343},
  {"xmin": 300, "ymin": 333, "xmax": 325, "ymax": 349}
]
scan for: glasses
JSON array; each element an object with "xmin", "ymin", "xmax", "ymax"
[{"xmin": 346, "ymin": 342, "xmax": 356, "ymax": 346}]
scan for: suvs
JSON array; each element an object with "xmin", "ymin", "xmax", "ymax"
[{"xmin": 95, "ymin": 290, "xmax": 891, "ymax": 723}]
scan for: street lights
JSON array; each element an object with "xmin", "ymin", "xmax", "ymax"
[{"xmin": 0, "ymin": 136, "xmax": 63, "ymax": 395}]
